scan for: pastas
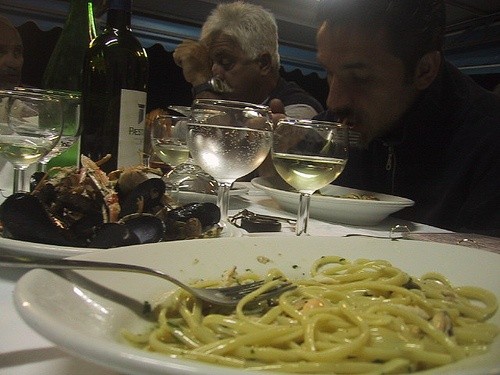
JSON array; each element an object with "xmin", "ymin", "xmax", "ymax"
[
  {"xmin": 119, "ymin": 256, "xmax": 500, "ymax": 375},
  {"xmin": 323, "ymin": 193, "xmax": 379, "ymax": 202}
]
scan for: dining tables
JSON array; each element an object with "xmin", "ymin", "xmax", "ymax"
[{"xmin": 0, "ymin": 181, "xmax": 500, "ymax": 375}]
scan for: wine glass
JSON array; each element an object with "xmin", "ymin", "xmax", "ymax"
[
  {"xmin": 151, "ymin": 99, "xmax": 274, "ymax": 239},
  {"xmin": 0, "ymin": 89, "xmax": 63, "ymax": 200},
  {"xmin": 270, "ymin": 118, "xmax": 350, "ymax": 237},
  {"xmin": 20, "ymin": 87, "xmax": 83, "ymax": 174}
]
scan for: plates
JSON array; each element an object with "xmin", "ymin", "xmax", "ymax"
[
  {"xmin": 10, "ymin": 231, "xmax": 500, "ymax": 375},
  {"xmin": 250, "ymin": 173, "xmax": 416, "ymax": 226},
  {"xmin": 0, "ymin": 206, "xmax": 248, "ymax": 262},
  {"xmin": 164, "ymin": 180, "xmax": 248, "ymax": 205}
]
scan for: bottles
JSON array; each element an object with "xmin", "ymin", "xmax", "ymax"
[
  {"xmin": 35, "ymin": 1, "xmax": 99, "ymax": 180},
  {"xmin": 84, "ymin": 1, "xmax": 151, "ymax": 184}
]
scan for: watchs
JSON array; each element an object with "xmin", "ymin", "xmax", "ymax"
[{"xmin": 193, "ymin": 76, "xmax": 225, "ymax": 94}]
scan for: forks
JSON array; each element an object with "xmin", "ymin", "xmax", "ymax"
[
  {"xmin": 0, "ymin": 257, "xmax": 295, "ymax": 311},
  {"xmin": 284, "ymin": 118, "xmax": 361, "ymax": 145}
]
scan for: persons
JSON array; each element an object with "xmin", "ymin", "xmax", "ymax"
[
  {"xmin": 0, "ymin": 16, "xmax": 31, "ymax": 89},
  {"xmin": 245, "ymin": 0, "xmax": 500, "ymax": 230},
  {"xmin": 145, "ymin": 0, "xmax": 323, "ymax": 163}
]
